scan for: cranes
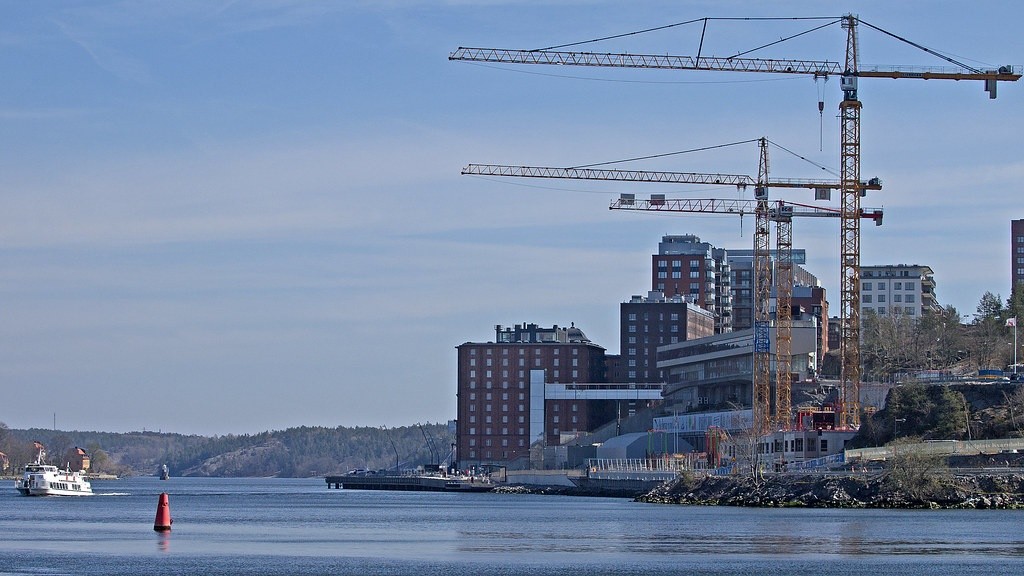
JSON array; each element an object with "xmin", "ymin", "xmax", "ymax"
[
  {"xmin": 458, "ymin": 136, "xmax": 881, "ymax": 434},
  {"xmin": 607, "ymin": 194, "xmax": 884, "ymax": 436},
  {"xmin": 450, "ymin": 13, "xmax": 1018, "ymax": 434}
]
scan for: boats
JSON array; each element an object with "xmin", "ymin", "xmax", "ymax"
[
  {"xmin": 160, "ymin": 464, "xmax": 169, "ymax": 480},
  {"xmin": 16, "ymin": 443, "xmax": 93, "ymax": 496}
]
